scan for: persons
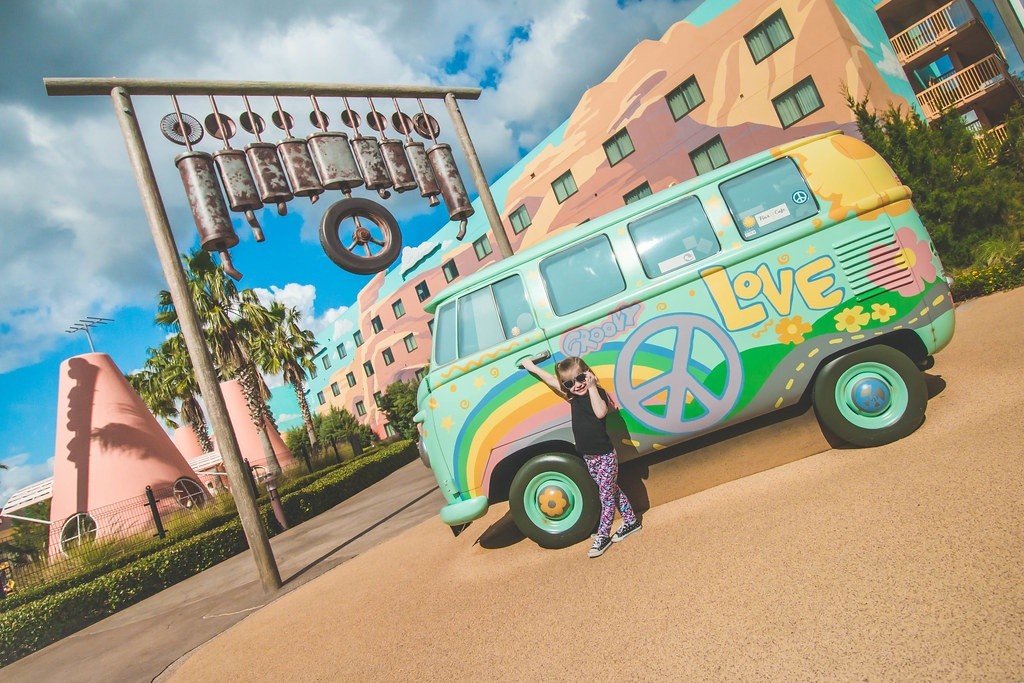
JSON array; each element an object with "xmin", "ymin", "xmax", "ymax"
[{"xmin": 522, "ymin": 355, "xmax": 643, "ymax": 559}]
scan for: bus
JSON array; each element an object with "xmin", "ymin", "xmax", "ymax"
[{"xmin": 410, "ymin": 128, "xmax": 957, "ymax": 550}]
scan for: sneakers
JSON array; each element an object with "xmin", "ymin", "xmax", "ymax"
[
  {"xmin": 610, "ymin": 519, "xmax": 642, "ymax": 543},
  {"xmin": 587, "ymin": 534, "xmax": 612, "ymax": 557}
]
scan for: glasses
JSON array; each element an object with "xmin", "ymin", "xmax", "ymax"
[{"xmin": 561, "ymin": 372, "xmax": 586, "ymax": 389}]
